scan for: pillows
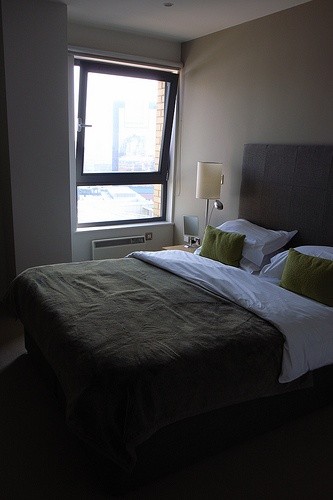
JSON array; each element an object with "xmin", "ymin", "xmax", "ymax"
[
  {"xmin": 279, "ymin": 248, "xmax": 333, "ymax": 308},
  {"xmin": 214, "ymin": 219, "xmax": 298, "ymax": 266},
  {"xmin": 199, "ymin": 225, "xmax": 246, "ymax": 268},
  {"xmin": 194, "ymin": 244, "xmax": 285, "ymax": 274},
  {"xmin": 259, "ymin": 245, "xmax": 333, "ymax": 279}
]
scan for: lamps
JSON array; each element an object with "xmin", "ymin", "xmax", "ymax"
[{"xmin": 196, "ymin": 161, "xmax": 223, "ymax": 230}]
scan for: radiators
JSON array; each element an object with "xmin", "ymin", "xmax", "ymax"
[{"xmin": 92, "ymin": 235, "xmax": 145, "ymax": 260}]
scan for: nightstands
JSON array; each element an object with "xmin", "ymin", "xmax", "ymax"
[{"xmin": 161, "ymin": 245, "xmax": 197, "ymax": 254}]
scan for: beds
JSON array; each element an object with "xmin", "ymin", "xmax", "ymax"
[{"xmin": 9, "ymin": 145, "xmax": 333, "ymax": 497}]
